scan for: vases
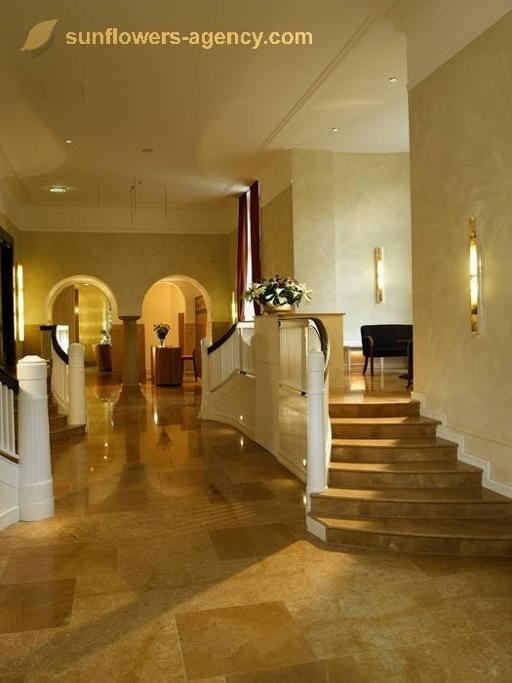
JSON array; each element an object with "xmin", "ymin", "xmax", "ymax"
[
  {"xmin": 160, "ymin": 339, "xmax": 163, "ymax": 346},
  {"xmin": 263, "ymin": 302, "xmax": 295, "ymax": 316}
]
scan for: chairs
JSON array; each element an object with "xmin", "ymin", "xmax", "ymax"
[
  {"xmin": 180, "ymin": 348, "xmax": 197, "ymax": 381},
  {"xmin": 361, "ymin": 324, "xmax": 413, "ymax": 374}
]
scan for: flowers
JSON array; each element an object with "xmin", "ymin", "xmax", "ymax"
[
  {"xmin": 153, "ymin": 322, "xmax": 171, "ymax": 339},
  {"xmin": 244, "ymin": 273, "xmax": 313, "ymax": 304}
]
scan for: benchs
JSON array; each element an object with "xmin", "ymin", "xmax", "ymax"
[{"xmin": 343, "ymin": 347, "xmax": 384, "ymax": 372}]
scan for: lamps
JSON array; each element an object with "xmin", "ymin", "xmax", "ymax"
[
  {"xmin": 467, "ymin": 214, "xmax": 482, "ymax": 336},
  {"xmin": 373, "ymin": 245, "xmax": 387, "ymax": 304}
]
